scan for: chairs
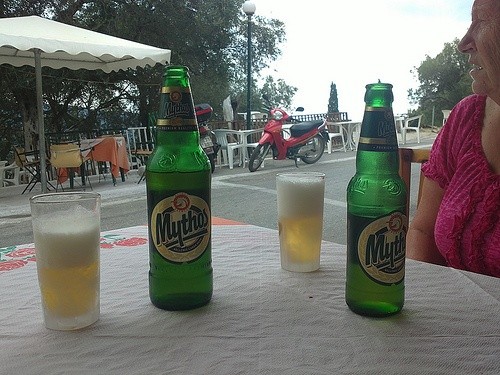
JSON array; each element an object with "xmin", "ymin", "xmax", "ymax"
[{"xmin": 14, "ymin": 111, "xmax": 430, "ymax": 228}]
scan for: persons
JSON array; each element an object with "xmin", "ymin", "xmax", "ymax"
[{"xmin": 405, "ymin": 0, "xmax": 500, "ymax": 279}]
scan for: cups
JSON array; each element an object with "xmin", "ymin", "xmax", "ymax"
[
  {"xmin": 275, "ymin": 171, "xmax": 325, "ymax": 273},
  {"xmin": 30, "ymin": 192, "xmax": 101, "ymax": 331}
]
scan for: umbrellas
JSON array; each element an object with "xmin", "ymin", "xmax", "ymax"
[{"xmin": 0, "ymin": 16, "xmax": 171, "ymax": 192}]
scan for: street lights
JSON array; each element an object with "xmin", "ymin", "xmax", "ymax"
[{"xmin": 242, "ymin": 0, "xmax": 257, "ymax": 160}]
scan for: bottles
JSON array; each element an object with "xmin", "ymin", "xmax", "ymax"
[
  {"xmin": 144, "ymin": 64, "xmax": 214, "ymax": 311},
  {"xmin": 344, "ymin": 82, "xmax": 410, "ymax": 318}
]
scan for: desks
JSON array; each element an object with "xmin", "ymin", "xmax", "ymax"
[
  {"xmin": 0, "ymin": 218, "xmax": 500, "ymax": 375},
  {"xmin": 57, "ymin": 137, "xmax": 130, "ymax": 189},
  {"xmin": 234, "ymin": 130, "xmax": 254, "ymax": 169},
  {"xmin": 337, "ymin": 120, "xmax": 362, "ymax": 151}
]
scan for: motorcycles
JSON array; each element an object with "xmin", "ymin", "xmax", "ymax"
[{"xmin": 248, "ymin": 94, "xmax": 330, "ymax": 172}]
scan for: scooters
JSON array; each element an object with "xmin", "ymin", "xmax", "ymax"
[{"xmin": 194, "ymin": 103, "xmax": 217, "ymax": 173}]
